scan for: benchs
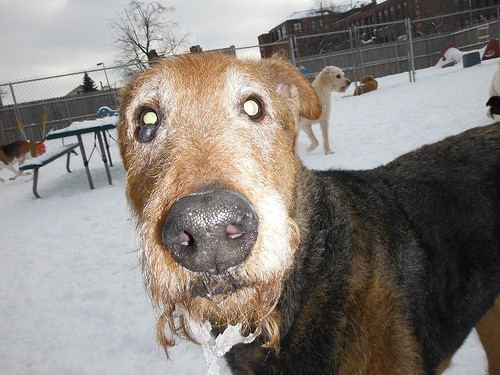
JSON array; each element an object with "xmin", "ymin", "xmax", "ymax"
[{"xmin": 19, "ymin": 143, "xmax": 80, "ymax": 198}]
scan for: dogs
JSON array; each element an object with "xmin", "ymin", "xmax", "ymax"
[
  {"xmin": 118, "ymin": 53, "xmax": 500, "ymax": 375},
  {"xmin": 0, "ymin": 136, "xmax": 48, "ymax": 183},
  {"xmin": 342, "ymin": 76, "xmax": 377, "ymax": 97},
  {"xmin": 297, "ymin": 66, "xmax": 352, "ymax": 155}
]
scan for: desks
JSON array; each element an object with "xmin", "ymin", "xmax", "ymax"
[{"xmin": 46, "ymin": 116, "xmax": 118, "ymax": 189}]
